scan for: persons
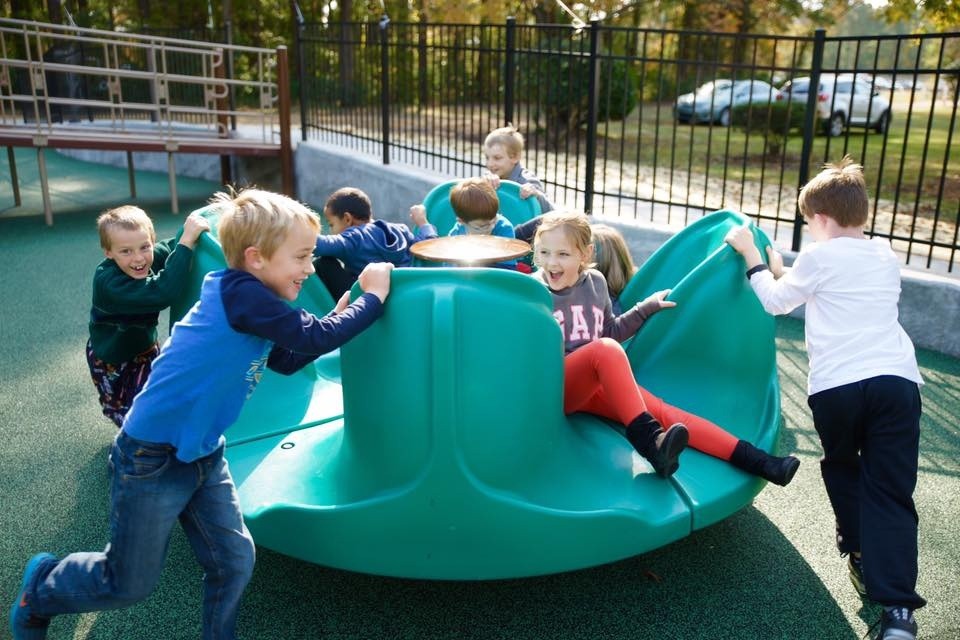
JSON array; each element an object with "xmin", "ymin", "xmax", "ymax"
[
  {"xmin": 312, "ymin": 187, "xmax": 437, "ymax": 304},
  {"xmin": 724, "ymin": 157, "xmax": 927, "ymax": 640},
  {"xmin": 443, "ymin": 177, "xmax": 518, "ymax": 271},
  {"xmin": 588, "ymin": 223, "xmax": 635, "ymax": 300},
  {"xmin": 483, "ymin": 126, "xmax": 554, "ymax": 213},
  {"xmin": 526, "ymin": 209, "xmax": 800, "ymax": 488},
  {"xmin": 8, "ymin": 187, "xmax": 395, "ymax": 640},
  {"xmin": 86, "ymin": 206, "xmax": 210, "ymax": 430}
]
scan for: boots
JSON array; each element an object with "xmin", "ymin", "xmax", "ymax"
[
  {"xmin": 626, "ymin": 409, "xmax": 691, "ymax": 480},
  {"xmin": 730, "ymin": 439, "xmax": 801, "ymax": 489}
]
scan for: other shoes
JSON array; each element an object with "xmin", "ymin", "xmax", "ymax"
[
  {"xmin": 863, "ymin": 602, "xmax": 917, "ymax": 640},
  {"xmin": 845, "ymin": 551, "xmax": 872, "ymax": 596}
]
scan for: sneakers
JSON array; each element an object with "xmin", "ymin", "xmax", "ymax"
[{"xmin": 8, "ymin": 551, "xmax": 57, "ymax": 640}]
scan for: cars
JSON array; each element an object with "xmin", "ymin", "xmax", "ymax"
[
  {"xmin": 673, "ymin": 78, "xmax": 781, "ymax": 126},
  {"xmin": 776, "ymin": 75, "xmax": 893, "ymax": 136},
  {"xmin": 855, "ymin": 72, "xmax": 923, "ymax": 91}
]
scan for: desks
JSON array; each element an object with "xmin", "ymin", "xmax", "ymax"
[{"xmin": 408, "ymin": 235, "xmax": 531, "ymax": 269}]
